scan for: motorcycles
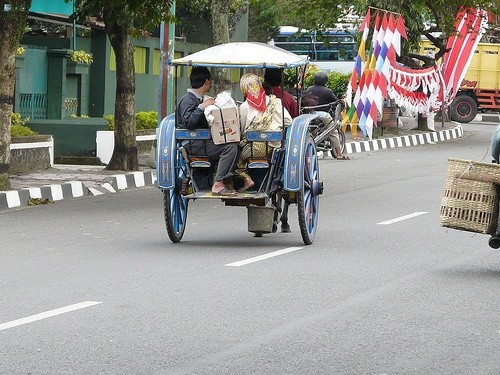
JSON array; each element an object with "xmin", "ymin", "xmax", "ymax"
[{"xmin": 300, "ymin": 100, "xmax": 345, "ymax": 158}]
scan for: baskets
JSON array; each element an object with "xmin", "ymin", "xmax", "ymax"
[{"xmin": 440, "ymin": 157, "xmax": 500, "ymax": 233}]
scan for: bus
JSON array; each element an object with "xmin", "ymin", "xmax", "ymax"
[{"xmin": 269, "ymin": 25, "xmax": 358, "ymax": 75}]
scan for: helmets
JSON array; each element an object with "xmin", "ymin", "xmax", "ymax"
[{"xmin": 314, "ymin": 72, "xmax": 328, "ymax": 86}]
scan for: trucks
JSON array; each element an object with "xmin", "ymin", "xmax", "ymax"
[{"xmin": 408, "ymin": 40, "xmax": 500, "ymax": 123}]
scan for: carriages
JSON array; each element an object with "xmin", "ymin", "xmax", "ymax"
[{"xmin": 155, "ymin": 42, "xmax": 340, "ymax": 245}]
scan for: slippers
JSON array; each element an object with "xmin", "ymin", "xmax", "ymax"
[
  {"xmin": 212, "ymin": 188, "xmax": 237, "ymax": 197},
  {"xmin": 337, "ymin": 155, "xmax": 350, "ymax": 160},
  {"xmin": 238, "ymin": 188, "xmax": 257, "ymax": 194}
]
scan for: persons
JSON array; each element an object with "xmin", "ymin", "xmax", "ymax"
[
  {"xmin": 261, "ymin": 67, "xmax": 300, "ymax": 121},
  {"xmin": 304, "ymin": 71, "xmax": 352, "ymax": 160},
  {"xmin": 488, "ymin": 113, "xmax": 500, "ymax": 249},
  {"xmin": 175, "ymin": 66, "xmax": 239, "ymax": 196},
  {"xmin": 233, "ymin": 72, "xmax": 293, "ymax": 194}
]
